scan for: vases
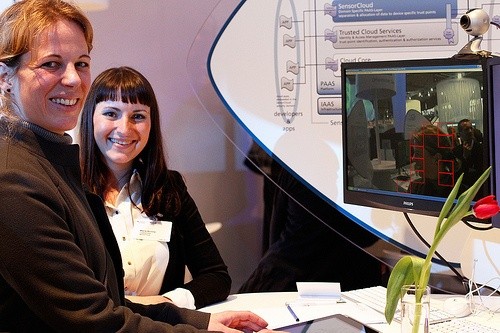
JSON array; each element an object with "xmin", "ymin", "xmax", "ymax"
[{"xmin": 400, "ymin": 285, "xmax": 430, "ymax": 333}]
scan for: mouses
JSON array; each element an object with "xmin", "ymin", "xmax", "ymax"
[{"xmin": 442, "ymin": 296, "xmax": 475, "ymax": 318}]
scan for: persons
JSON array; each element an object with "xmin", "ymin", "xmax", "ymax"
[
  {"xmin": 369, "ymin": 118, "xmax": 483, "ymax": 194},
  {"xmin": 270, "ymin": 158, "xmax": 381, "ymax": 293},
  {"xmin": 0, "ymin": 1, "xmax": 281, "ymax": 333},
  {"xmin": 78, "ymin": 66, "xmax": 232, "ymax": 313}
]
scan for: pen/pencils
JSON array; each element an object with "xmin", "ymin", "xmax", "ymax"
[{"xmin": 285, "ymin": 303, "xmax": 301, "ymax": 324}]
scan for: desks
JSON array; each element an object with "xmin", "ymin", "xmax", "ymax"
[{"xmin": 185, "ymin": 274, "xmax": 499, "ymax": 333}]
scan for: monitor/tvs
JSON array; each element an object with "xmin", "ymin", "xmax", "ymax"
[{"xmin": 341, "ymin": 55, "xmax": 500, "ymax": 224}]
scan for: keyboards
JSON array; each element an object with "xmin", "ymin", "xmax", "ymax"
[
  {"xmin": 340, "ymin": 286, "xmax": 455, "ymax": 326},
  {"xmin": 428, "ymin": 319, "xmax": 500, "ymax": 333}
]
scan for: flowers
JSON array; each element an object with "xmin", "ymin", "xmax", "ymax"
[{"xmin": 385, "ymin": 165, "xmax": 500, "ymax": 333}]
